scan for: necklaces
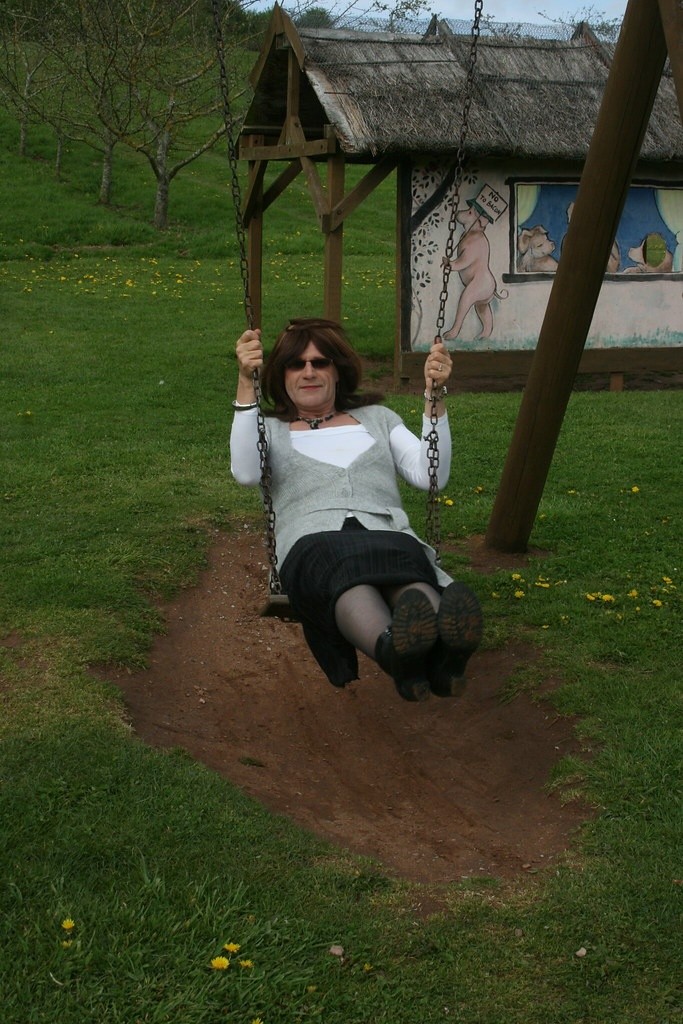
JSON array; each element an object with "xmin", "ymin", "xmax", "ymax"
[{"xmin": 298, "ymin": 412, "xmax": 337, "ymax": 430}]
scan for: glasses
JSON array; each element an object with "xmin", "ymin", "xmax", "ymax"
[{"xmin": 284, "ymin": 356, "xmax": 333, "ymax": 371}]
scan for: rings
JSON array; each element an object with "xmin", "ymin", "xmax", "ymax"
[{"xmin": 438, "ymin": 362, "xmax": 443, "ymax": 371}]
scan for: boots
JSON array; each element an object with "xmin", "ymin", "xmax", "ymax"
[
  {"xmin": 374, "ymin": 588, "xmax": 436, "ymax": 702},
  {"xmin": 429, "ymin": 581, "xmax": 483, "ymax": 697}
]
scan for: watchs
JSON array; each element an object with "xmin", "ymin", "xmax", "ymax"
[{"xmin": 424, "ymin": 386, "xmax": 448, "ymax": 402}]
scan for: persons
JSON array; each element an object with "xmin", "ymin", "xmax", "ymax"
[{"xmin": 231, "ymin": 319, "xmax": 485, "ymax": 701}]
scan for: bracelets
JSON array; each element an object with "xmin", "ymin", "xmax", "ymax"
[{"xmin": 231, "ymin": 399, "xmax": 259, "ymax": 411}]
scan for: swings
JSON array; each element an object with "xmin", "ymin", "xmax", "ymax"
[{"xmin": 203, "ymin": 0, "xmax": 482, "ymax": 686}]
